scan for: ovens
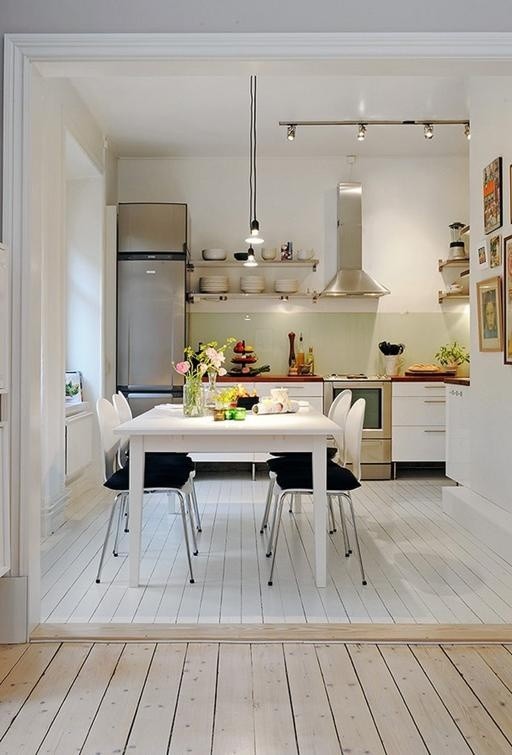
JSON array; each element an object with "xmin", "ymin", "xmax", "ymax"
[{"xmin": 322, "ymin": 373, "xmax": 392, "ymax": 464}]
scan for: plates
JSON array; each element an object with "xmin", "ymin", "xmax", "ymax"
[
  {"xmin": 274, "ymin": 279, "xmax": 298, "ymax": 294},
  {"xmin": 202, "ymin": 248, "xmax": 226, "ymax": 260},
  {"xmin": 239, "ymin": 274, "xmax": 265, "ymax": 294},
  {"xmin": 199, "ymin": 276, "xmax": 230, "ymax": 294}
]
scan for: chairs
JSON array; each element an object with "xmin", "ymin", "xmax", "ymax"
[
  {"xmin": 117, "ymin": 390, "xmax": 196, "ymax": 515},
  {"xmin": 259, "ymin": 389, "xmax": 354, "ymax": 535},
  {"xmin": 266, "ymin": 397, "xmax": 368, "ymax": 587},
  {"xmin": 95, "ymin": 397, "xmax": 200, "ymax": 584},
  {"xmin": 112, "ymin": 393, "xmax": 204, "ymax": 533}
]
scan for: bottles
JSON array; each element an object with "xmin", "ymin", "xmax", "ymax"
[
  {"xmin": 288, "ymin": 353, "xmax": 298, "ymax": 375},
  {"xmin": 306, "ymin": 347, "xmax": 314, "ymax": 375},
  {"xmin": 296, "ymin": 336, "xmax": 304, "ymax": 364}
]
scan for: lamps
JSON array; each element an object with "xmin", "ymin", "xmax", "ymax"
[
  {"xmin": 464, "ymin": 124, "xmax": 470, "ymax": 139},
  {"xmin": 357, "ymin": 123, "xmax": 368, "ymax": 142},
  {"xmin": 244, "ymin": 75, "xmax": 265, "ymax": 245},
  {"xmin": 423, "ymin": 122, "xmax": 435, "ymax": 141},
  {"xmin": 286, "ymin": 123, "xmax": 296, "ymax": 142},
  {"xmin": 243, "ymin": 74, "xmax": 258, "ymax": 268}
]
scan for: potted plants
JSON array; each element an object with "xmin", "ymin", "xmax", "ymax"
[{"xmin": 435, "ymin": 341, "xmax": 470, "ymax": 374}]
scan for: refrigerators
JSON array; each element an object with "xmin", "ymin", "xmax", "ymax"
[{"xmin": 115, "ymin": 202, "xmax": 188, "ymax": 420}]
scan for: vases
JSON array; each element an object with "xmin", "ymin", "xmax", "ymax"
[
  {"xmin": 181, "ymin": 380, "xmax": 205, "ymax": 419},
  {"xmin": 200, "ymin": 371, "xmax": 229, "ymax": 410}
]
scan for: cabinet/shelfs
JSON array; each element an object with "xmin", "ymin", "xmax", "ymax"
[
  {"xmin": 64, "ymin": 410, "xmax": 94, "ymax": 477},
  {"xmin": 437, "ymin": 258, "xmax": 469, "ymax": 272},
  {"xmin": 182, "ymin": 383, "xmax": 325, "ymax": 462},
  {"xmin": 439, "ymin": 290, "xmax": 469, "ymax": 305},
  {"xmin": 187, "ymin": 259, "xmax": 320, "ymax": 272},
  {"xmin": 391, "ymin": 382, "xmax": 445, "ymax": 463},
  {"xmin": 187, "ymin": 290, "xmax": 318, "ymax": 306}
]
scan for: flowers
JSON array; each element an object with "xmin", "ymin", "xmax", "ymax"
[
  {"xmin": 183, "ymin": 335, "xmax": 238, "ymax": 394},
  {"xmin": 171, "ymin": 337, "xmax": 236, "ymax": 413}
]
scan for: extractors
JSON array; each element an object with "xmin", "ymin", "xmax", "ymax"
[{"xmin": 318, "ymin": 181, "xmax": 390, "ymax": 299}]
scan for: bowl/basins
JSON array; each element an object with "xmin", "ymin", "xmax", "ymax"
[
  {"xmin": 296, "ymin": 247, "xmax": 315, "ymax": 261},
  {"xmin": 261, "ymin": 246, "xmax": 277, "ymax": 262},
  {"xmin": 234, "ymin": 253, "xmax": 250, "ymax": 261}
]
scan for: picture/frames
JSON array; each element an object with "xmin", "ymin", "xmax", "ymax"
[
  {"xmin": 482, "ymin": 156, "xmax": 503, "ymax": 235},
  {"xmin": 503, "ymin": 234, "xmax": 512, "ymax": 365},
  {"xmin": 476, "ymin": 276, "xmax": 502, "ymax": 352}
]
